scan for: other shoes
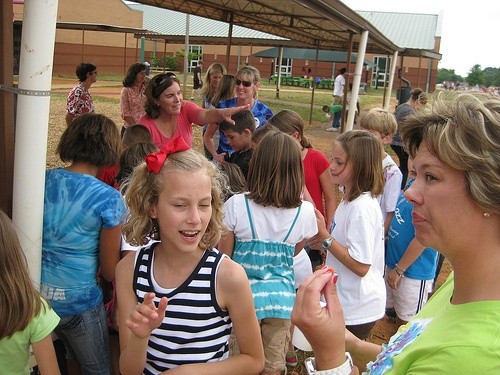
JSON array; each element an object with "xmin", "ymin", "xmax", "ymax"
[
  {"xmin": 293, "ymin": 346, "xmax": 299, "ymax": 352},
  {"xmin": 336, "ymin": 127, "xmax": 340, "ymax": 131},
  {"xmin": 326, "ymin": 127, "xmax": 336, "ymax": 131},
  {"xmin": 285, "ymin": 354, "xmax": 297, "ymax": 366}
]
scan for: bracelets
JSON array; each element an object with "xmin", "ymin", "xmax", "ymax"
[{"xmin": 395, "ymin": 264, "xmax": 406, "ymax": 277}]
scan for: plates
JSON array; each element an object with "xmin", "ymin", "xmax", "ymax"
[{"xmin": 291, "ymin": 300, "xmax": 327, "ymax": 353}]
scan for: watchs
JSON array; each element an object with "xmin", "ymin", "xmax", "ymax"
[
  {"xmin": 304, "ymin": 352, "xmax": 354, "ymax": 375},
  {"xmin": 323, "ymin": 236, "xmax": 335, "ymax": 249}
]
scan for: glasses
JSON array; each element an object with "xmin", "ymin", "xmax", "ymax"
[
  {"xmin": 236, "ymin": 79, "xmax": 255, "ymax": 87},
  {"xmin": 88, "ymin": 72, "xmax": 97, "ymax": 75},
  {"xmin": 153, "ymin": 73, "xmax": 177, "ymax": 88}
]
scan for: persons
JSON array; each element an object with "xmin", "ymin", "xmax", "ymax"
[
  {"xmin": 359, "ymin": 108, "xmax": 403, "ymax": 231},
  {"xmin": 323, "ymin": 104, "xmax": 342, "ymax": 131},
  {"xmin": 390, "ymin": 88, "xmax": 428, "ymax": 190},
  {"xmin": 40, "ymin": 112, "xmax": 128, "ymax": 375},
  {"xmin": 301, "ymin": 67, "xmax": 312, "ymax": 78},
  {"xmin": 481, "ymin": 85, "xmax": 495, "ymax": 95},
  {"xmin": 120, "ymin": 63, "xmax": 273, "ymax": 197},
  {"xmin": 307, "ymin": 130, "xmax": 386, "ymax": 339},
  {"xmin": 65, "ymin": 64, "xmax": 98, "ymax": 126},
  {"xmin": 0, "ymin": 209, "xmax": 60, "ymax": 375},
  {"xmin": 442, "ymin": 81, "xmax": 469, "ymax": 90},
  {"xmin": 100, "ymin": 124, "xmax": 161, "ymax": 256},
  {"xmin": 333, "ymin": 68, "xmax": 346, "ymax": 105},
  {"xmin": 345, "ymin": 83, "xmax": 361, "ymax": 125},
  {"xmin": 218, "ymin": 131, "xmax": 318, "ymax": 375},
  {"xmin": 384, "ymin": 154, "xmax": 438, "ymax": 333},
  {"xmin": 269, "ymin": 109, "xmax": 336, "ymax": 271},
  {"xmin": 220, "ymin": 110, "xmax": 254, "ymax": 181},
  {"xmin": 191, "ymin": 60, "xmax": 203, "ymax": 101},
  {"xmin": 252, "ymin": 124, "xmax": 316, "ymax": 365},
  {"xmin": 115, "ymin": 139, "xmax": 265, "ymax": 375},
  {"xmin": 291, "ymin": 86, "xmax": 500, "ymax": 375}
]
